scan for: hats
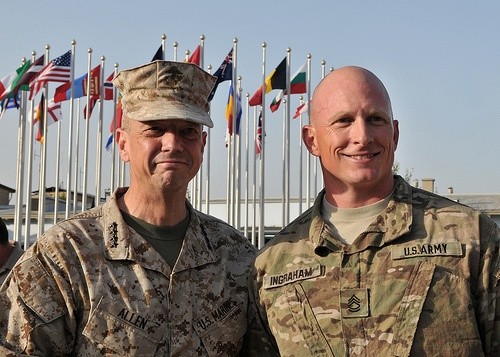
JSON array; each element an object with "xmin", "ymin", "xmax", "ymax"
[{"xmin": 111, "ymin": 60, "xmax": 218, "ymax": 128}]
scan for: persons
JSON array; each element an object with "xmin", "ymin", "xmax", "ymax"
[
  {"xmin": 246, "ymin": 66, "xmax": 500, "ymax": 357},
  {"xmin": 0, "ymin": 216, "xmax": 28, "ymax": 287},
  {"xmin": 0, "ymin": 60, "xmax": 270, "ymax": 357}
]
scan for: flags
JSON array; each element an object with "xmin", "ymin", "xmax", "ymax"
[{"xmin": 0, "ymin": 44, "xmax": 316, "ymax": 155}]
